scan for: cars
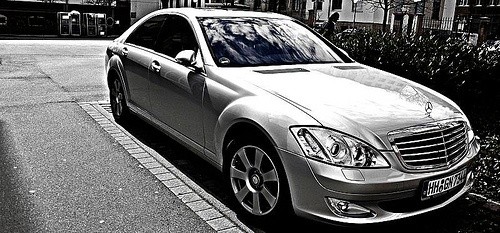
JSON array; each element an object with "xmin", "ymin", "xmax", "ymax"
[{"xmin": 104, "ymin": 7, "xmax": 480, "ymax": 225}]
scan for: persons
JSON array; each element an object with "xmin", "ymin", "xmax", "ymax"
[{"xmin": 326, "ymin": 12, "xmax": 341, "ymax": 31}]
